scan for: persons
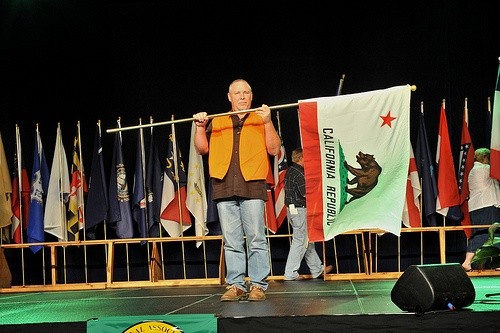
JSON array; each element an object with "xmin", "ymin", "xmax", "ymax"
[
  {"xmin": 193, "ymin": 79, "xmax": 281, "ymax": 302},
  {"xmin": 462, "ymin": 148, "xmax": 500, "ymax": 272},
  {"xmin": 283, "ymin": 149, "xmax": 333, "ymax": 281}
]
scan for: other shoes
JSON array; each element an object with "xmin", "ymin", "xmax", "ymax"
[
  {"xmin": 294, "ymin": 276, "xmax": 304, "ymax": 280},
  {"xmin": 320, "ymin": 265, "xmax": 333, "ymax": 276}
]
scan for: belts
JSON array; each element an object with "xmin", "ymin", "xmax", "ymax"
[{"xmin": 295, "ymin": 205, "xmax": 306, "ymax": 208}]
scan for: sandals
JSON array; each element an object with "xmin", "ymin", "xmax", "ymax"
[{"xmin": 463, "ymin": 265, "xmax": 474, "ymax": 272}]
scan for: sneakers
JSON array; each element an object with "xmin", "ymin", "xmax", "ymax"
[
  {"xmin": 248, "ymin": 286, "xmax": 266, "ymax": 301},
  {"xmin": 220, "ymin": 286, "xmax": 246, "ymax": 302}
]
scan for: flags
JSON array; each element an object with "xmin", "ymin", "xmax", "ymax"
[
  {"xmin": 265, "ymin": 127, "xmax": 290, "ymax": 234},
  {"xmin": 298, "ymin": 85, "xmax": 410, "ymax": 244},
  {"xmin": 402, "ymin": 63, "xmax": 500, "ymax": 239},
  {"xmin": 0, "ymin": 121, "xmax": 218, "ymax": 255}
]
